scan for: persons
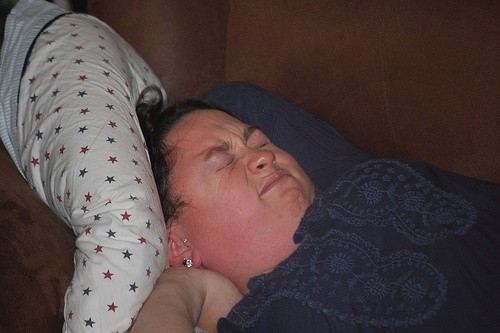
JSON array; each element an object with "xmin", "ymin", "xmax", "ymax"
[
  {"xmin": 118, "ymin": 80, "xmax": 499, "ymax": 333},
  {"xmin": 0, "ymin": 0, "xmax": 169, "ymax": 333}
]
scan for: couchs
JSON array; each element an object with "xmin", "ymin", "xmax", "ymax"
[{"xmin": 55, "ymin": 0, "xmax": 500, "ymax": 185}]
